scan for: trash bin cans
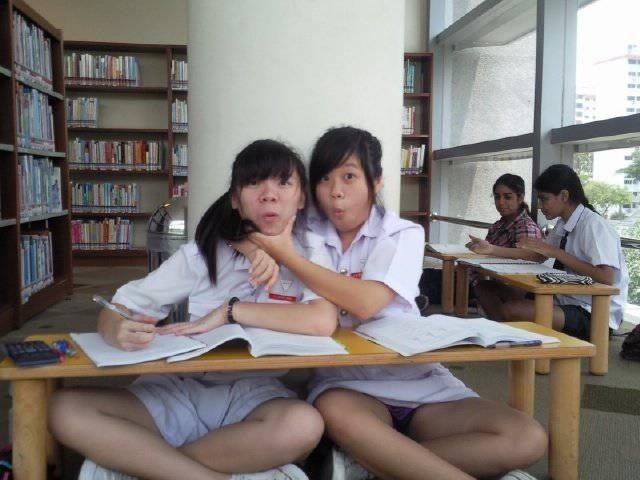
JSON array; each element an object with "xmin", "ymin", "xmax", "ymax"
[{"xmin": 145, "ymin": 196, "xmax": 188, "ymax": 327}]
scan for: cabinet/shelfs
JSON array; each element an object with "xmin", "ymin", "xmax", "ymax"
[
  {"xmin": 402, "ymin": 52, "xmax": 433, "ymax": 220},
  {"xmin": 172, "ymin": 45, "xmax": 187, "ymax": 197},
  {"xmin": 65, "ymin": 42, "xmax": 170, "ymax": 265},
  {"xmin": 2, "ymin": 1, "xmax": 74, "ymax": 335}
]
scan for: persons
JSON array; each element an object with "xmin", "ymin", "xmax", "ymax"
[
  {"xmin": 473, "ymin": 171, "xmax": 545, "ymax": 317},
  {"xmin": 49, "ymin": 138, "xmax": 340, "ymax": 480},
  {"xmin": 465, "ymin": 164, "xmax": 631, "ymax": 339},
  {"xmin": 228, "ymin": 125, "xmax": 548, "ymax": 480}
]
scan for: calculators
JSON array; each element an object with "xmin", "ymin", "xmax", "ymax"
[{"xmin": 3, "ymin": 341, "xmax": 58, "ymax": 366}]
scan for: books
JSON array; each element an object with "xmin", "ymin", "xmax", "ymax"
[
  {"xmin": 62, "ymin": 52, "xmax": 190, "ymax": 250},
  {"xmin": 400, "ymin": 59, "xmax": 425, "ymax": 176},
  {"xmin": 10, "ymin": 10, "xmax": 62, "ymax": 304},
  {"xmin": 70, "ymin": 322, "xmax": 349, "ymax": 368},
  {"xmin": 427, "ymin": 244, "xmax": 565, "ymax": 275},
  {"xmin": 351, "ymin": 314, "xmax": 560, "ymax": 357}
]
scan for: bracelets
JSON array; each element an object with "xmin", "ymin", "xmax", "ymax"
[{"xmin": 226, "ymin": 296, "xmax": 241, "ymax": 323}]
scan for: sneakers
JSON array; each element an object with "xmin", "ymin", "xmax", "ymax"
[
  {"xmin": 78, "ymin": 459, "xmax": 140, "ymax": 480},
  {"xmin": 233, "ymin": 463, "xmax": 308, "ymax": 480},
  {"xmin": 332, "ymin": 451, "xmax": 370, "ymax": 480}
]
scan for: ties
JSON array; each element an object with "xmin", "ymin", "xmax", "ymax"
[{"xmin": 553, "ymin": 234, "xmax": 568, "ymax": 270}]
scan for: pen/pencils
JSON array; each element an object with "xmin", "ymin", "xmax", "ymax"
[
  {"xmin": 91, "ymin": 295, "xmax": 134, "ymax": 322},
  {"xmin": 486, "ymin": 340, "xmax": 542, "ymax": 349}
]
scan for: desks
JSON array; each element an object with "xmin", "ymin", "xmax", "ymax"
[
  {"xmin": 1, "ymin": 319, "xmax": 599, "ymax": 480},
  {"xmin": 421, "ymin": 245, "xmax": 620, "ymax": 376}
]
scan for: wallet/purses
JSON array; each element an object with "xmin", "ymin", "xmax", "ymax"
[{"xmin": 538, "ymin": 274, "xmax": 594, "ymax": 285}]
